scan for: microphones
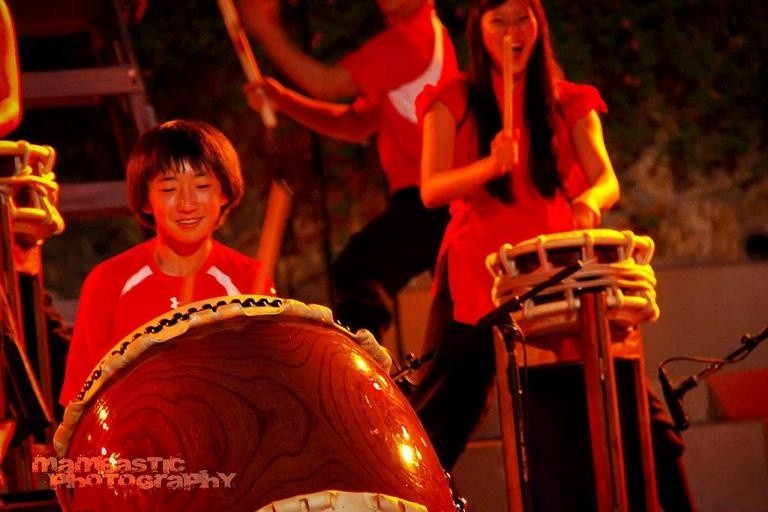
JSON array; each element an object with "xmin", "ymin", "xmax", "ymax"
[{"xmin": 658, "ymin": 368, "xmax": 691, "ymax": 433}]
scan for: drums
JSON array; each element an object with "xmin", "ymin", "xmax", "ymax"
[
  {"xmin": 484, "ymin": 228, "xmax": 661, "ymax": 340},
  {"xmin": 52, "ymin": 294, "xmax": 470, "ymax": 511},
  {"xmin": 2, "ymin": 141, "xmax": 66, "ymax": 243}
]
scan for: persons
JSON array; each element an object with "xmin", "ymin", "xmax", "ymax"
[
  {"xmin": 417, "ymin": 0, "xmax": 693, "ymax": 511},
  {"xmin": 0, "ymin": 2, "xmax": 71, "ymax": 439},
  {"xmin": 59, "ymin": 120, "xmax": 275, "ymax": 407},
  {"xmin": 241, "ymin": 0, "xmax": 481, "ymax": 341}
]
